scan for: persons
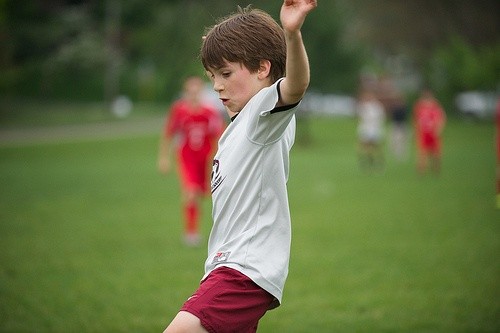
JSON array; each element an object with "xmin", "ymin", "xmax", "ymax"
[
  {"xmin": 159, "ymin": 72, "xmax": 226, "ymax": 246},
  {"xmin": 354, "ymin": 85, "xmax": 386, "ymax": 177},
  {"xmin": 163, "ymin": 0, "xmax": 319, "ymax": 333},
  {"xmin": 413, "ymin": 85, "xmax": 448, "ymax": 188},
  {"xmin": 380, "ymin": 83, "xmax": 412, "ymax": 159}
]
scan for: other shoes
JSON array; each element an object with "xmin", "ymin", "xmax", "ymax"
[{"xmin": 186, "ymin": 232, "xmax": 200, "ymax": 245}]
